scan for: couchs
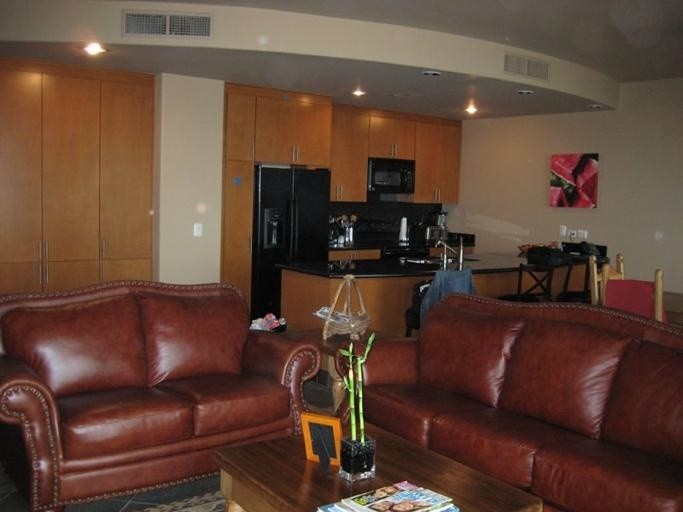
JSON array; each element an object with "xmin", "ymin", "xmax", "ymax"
[
  {"xmin": 0, "ymin": 276, "xmax": 322, "ymax": 511},
  {"xmin": 323, "ymin": 290, "xmax": 681, "ymax": 512}
]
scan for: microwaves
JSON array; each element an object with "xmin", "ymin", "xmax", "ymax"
[{"xmin": 367, "ymin": 158, "xmax": 414, "ymax": 193}]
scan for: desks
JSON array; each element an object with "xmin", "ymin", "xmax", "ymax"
[
  {"xmin": 662, "ymin": 289, "xmax": 683, "ymax": 326},
  {"xmin": 279, "ymin": 327, "xmax": 397, "ymax": 420}
]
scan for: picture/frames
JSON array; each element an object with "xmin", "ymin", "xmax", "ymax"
[{"xmin": 299, "ymin": 409, "xmax": 342, "ymax": 470}]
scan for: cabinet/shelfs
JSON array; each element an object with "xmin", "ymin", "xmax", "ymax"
[
  {"xmin": 330, "ymin": 99, "xmax": 370, "ymax": 203},
  {"xmin": 369, "ymin": 108, "xmax": 416, "ymax": 161},
  {"xmin": 427, "ymin": 243, "xmax": 477, "ymax": 257},
  {"xmin": 255, "ymin": 85, "xmax": 335, "ymax": 169},
  {"xmin": 326, "ymin": 245, "xmax": 382, "ymax": 262},
  {"xmin": 0, "ymin": 56, "xmax": 155, "ymax": 299},
  {"xmin": 220, "ymin": 77, "xmax": 256, "ymax": 314},
  {"xmin": 394, "ymin": 112, "xmax": 464, "ymax": 206}
]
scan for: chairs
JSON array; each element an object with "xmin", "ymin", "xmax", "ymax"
[
  {"xmin": 402, "ymin": 266, "xmax": 472, "ymax": 337},
  {"xmin": 585, "ymin": 251, "xmax": 626, "ymax": 306},
  {"xmin": 598, "ymin": 261, "xmax": 670, "ymax": 324},
  {"xmin": 556, "ymin": 252, "xmax": 611, "ymax": 304},
  {"xmin": 492, "ymin": 260, "xmax": 555, "ymax": 304}
]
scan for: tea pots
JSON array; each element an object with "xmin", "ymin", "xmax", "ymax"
[{"xmin": 344, "ymin": 224, "xmax": 358, "ymax": 246}]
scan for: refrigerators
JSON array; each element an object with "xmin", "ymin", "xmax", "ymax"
[{"xmin": 250, "ymin": 162, "xmax": 330, "ymax": 325}]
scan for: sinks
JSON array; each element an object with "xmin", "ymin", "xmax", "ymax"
[{"xmin": 403, "ymin": 256, "xmax": 478, "ymax": 264}]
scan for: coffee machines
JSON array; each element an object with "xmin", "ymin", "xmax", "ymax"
[{"xmin": 434, "ymin": 212, "xmax": 447, "ymax": 227}]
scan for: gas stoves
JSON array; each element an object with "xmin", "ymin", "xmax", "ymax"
[{"xmin": 362, "ymin": 240, "xmax": 427, "ymax": 255}]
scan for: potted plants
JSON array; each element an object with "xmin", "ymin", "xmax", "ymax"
[{"xmin": 333, "ymin": 327, "xmax": 380, "ymax": 483}]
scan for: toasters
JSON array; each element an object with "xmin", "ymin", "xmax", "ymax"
[{"xmin": 423, "ymin": 225, "xmax": 448, "ymax": 243}]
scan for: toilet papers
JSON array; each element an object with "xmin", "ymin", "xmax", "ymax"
[{"xmin": 399, "ymin": 218, "xmax": 407, "ymax": 240}]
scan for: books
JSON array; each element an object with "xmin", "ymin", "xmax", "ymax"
[{"xmin": 313, "ymin": 479, "xmax": 462, "ymax": 512}]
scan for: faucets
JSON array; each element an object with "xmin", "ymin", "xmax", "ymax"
[{"xmin": 434, "ymin": 236, "xmax": 464, "ymax": 272}]
